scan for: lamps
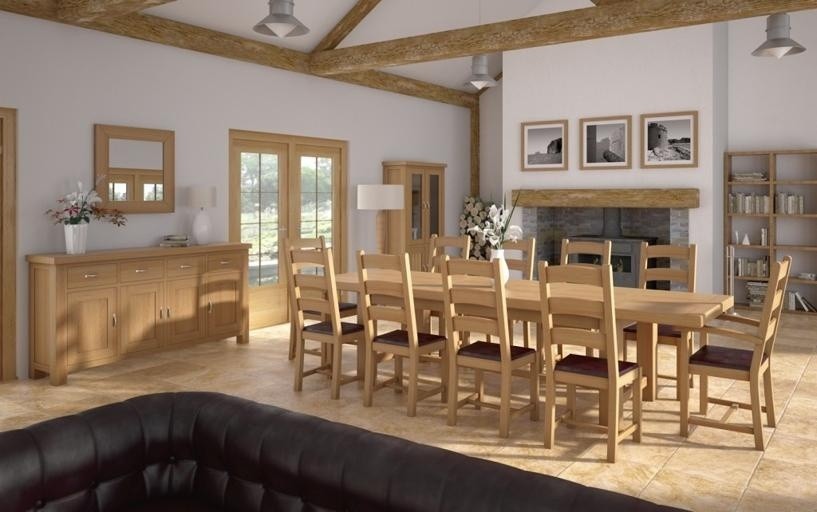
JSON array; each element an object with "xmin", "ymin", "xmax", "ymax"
[
  {"xmin": 464, "ymin": 54, "xmax": 502, "ymax": 90},
  {"xmin": 751, "ymin": 12, "xmax": 808, "ymax": 60},
  {"xmin": 186, "ymin": 186, "xmax": 218, "ymax": 245},
  {"xmin": 252, "ymin": 0, "xmax": 316, "ymax": 38},
  {"xmin": 357, "ymin": 185, "xmax": 405, "ymax": 255}
]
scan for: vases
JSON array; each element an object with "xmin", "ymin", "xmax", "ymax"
[{"xmin": 64, "ymin": 224, "xmax": 88, "ymax": 255}]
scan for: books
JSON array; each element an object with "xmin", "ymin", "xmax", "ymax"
[
  {"xmin": 728, "ymin": 171, "xmax": 807, "ymax": 216},
  {"xmin": 728, "ymin": 225, "xmax": 816, "ymax": 313}
]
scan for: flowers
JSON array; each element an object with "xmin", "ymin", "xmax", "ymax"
[
  {"xmin": 44, "ymin": 174, "xmax": 128, "ymax": 227},
  {"xmin": 467, "ymin": 190, "xmax": 523, "ymax": 260}
]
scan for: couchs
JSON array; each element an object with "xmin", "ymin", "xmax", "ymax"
[{"xmin": 0, "ymin": 392, "xmax": 689, "ymax": 512}]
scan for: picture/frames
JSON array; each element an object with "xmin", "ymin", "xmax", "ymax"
[
  {"xmin": 521, "ymin": 120, "xmax": 568, "ymax": 171},
  {"xmin": 579, "ymin": 115, "xmax": 633, "ymax": 170},
  {"xmin": 640, "ymin": 110, "xmax": 699, "ymax": 169}
]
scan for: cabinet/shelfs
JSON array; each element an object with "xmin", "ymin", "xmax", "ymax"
[
  {"xmin": 723, "ymin": 150, "xmax": 816, "ymax": 311},
  {"xmin": 26, "ymin": 243, "xmax": 252, "ymax": 387},
  {"xmin": 382, "ymin": 161, "xmax": 448, "ymax": 271}
]
